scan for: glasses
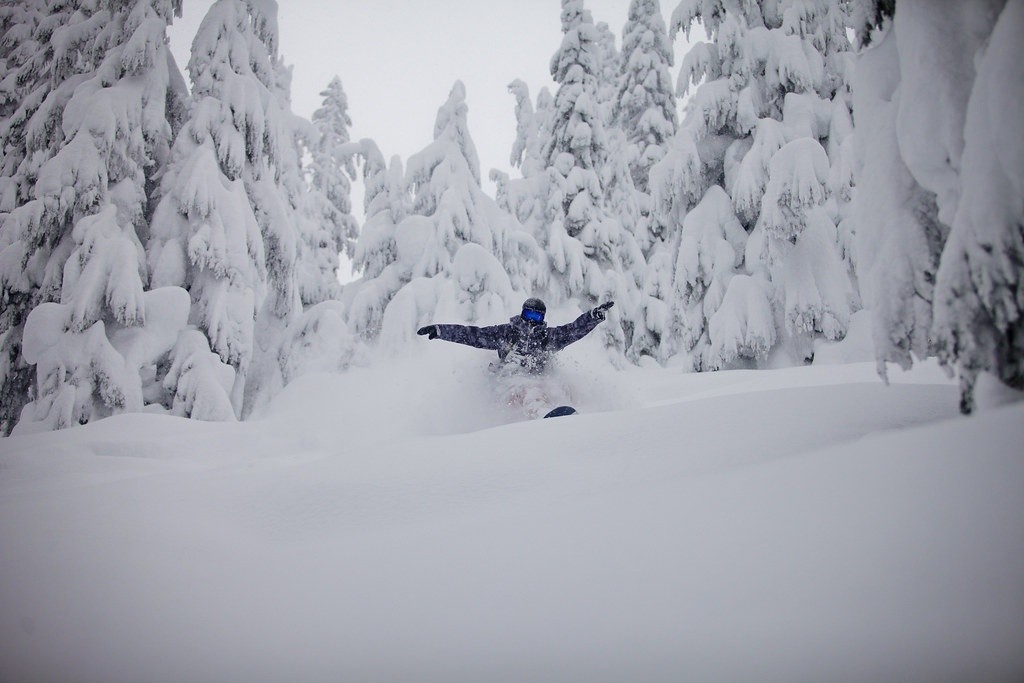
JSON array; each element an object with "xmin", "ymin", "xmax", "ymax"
[{"xmin": 521, "ymin": 309, "xmax": 545, "ymax": 325}]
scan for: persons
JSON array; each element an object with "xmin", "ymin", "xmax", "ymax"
[{"xmin": 417, "ymin": 298, "xmax": 614, "ymax": 419}]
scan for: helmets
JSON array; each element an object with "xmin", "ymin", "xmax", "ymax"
[{"xmin": 521, "ymin": 297, "xmax": 547, "ymax": 322}]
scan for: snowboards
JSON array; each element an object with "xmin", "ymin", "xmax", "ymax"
[{"xmin": 544, "ymin": 406, "xmax": 578, "ymax": 418}]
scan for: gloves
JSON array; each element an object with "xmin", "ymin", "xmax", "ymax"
[
  {"xmin": 416, "ymin": 326, "xmax": 437, "ymax": 340},
  {"xmin": 594, "ymin": 301, "xmax": 615, "ymax": 312}
]
